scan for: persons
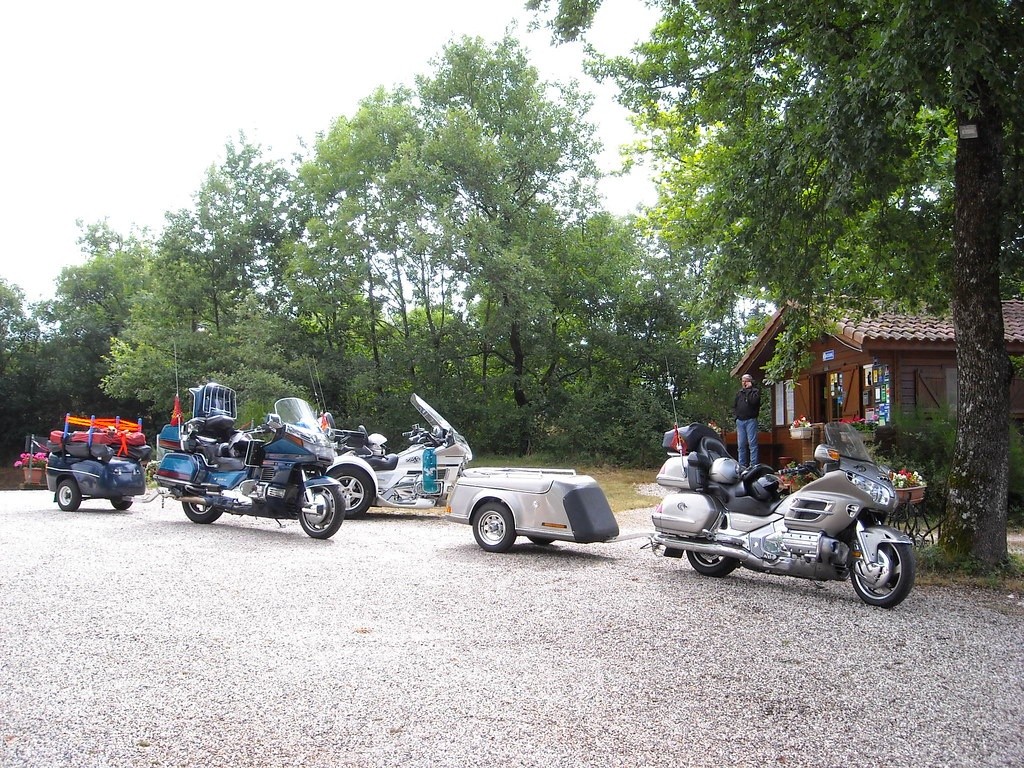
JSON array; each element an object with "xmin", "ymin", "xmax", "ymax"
[{"xmin": 731, "ymin": 373, "xmax": 762, "ymax": 468}]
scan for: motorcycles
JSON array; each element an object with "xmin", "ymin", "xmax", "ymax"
[
  {"xmin": 320, "ymin": 393, "xmax": 474, "ymax": 519},
  {"xmin": 647, "ymin": 419, "xmax": 915, "ymax": 606},
  {"xmin": 155, "ymin": 383, "xmax": 345, "ymax": 540}
]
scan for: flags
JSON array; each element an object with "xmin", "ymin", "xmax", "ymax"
[{"xmin": 669, "ymin": 422, "xmax": 687, "ymax": 457}]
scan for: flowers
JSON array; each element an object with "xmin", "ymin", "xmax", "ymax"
[
  {"xmin": 889, "ymin": 469, "xmax": 925, "ymax": 488},
  {"xmin": 790, "ymin": 415, "xmax": 810, "ymax": 428}
]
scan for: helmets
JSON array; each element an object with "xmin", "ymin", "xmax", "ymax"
[
  {"xmin": 368, "ymin": 433, "xmax": 387, "ymax": 456},
  {"xmin": 710, "ymin": 457, "xmax": 740, "ymax": 483},
  {"xmin": 748, "ymin": 472, "xmax": 785, "ymax": 501},
  {"xmin": 229, "ymin": 433, "xmax": 253, "ymax": 458}
]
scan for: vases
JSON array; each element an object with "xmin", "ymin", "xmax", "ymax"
[
  {"xmin": 894, "ymin": 485, "xmax": 925, "ymax": 503},
  {"xmin": 789, "ymin": 428, "xmax": 811, "ymax": 439}
]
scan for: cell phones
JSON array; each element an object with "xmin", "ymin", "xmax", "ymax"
[{"xmin": 745, "ymin": 381, "xmax": 754, "ymax": 388}]
